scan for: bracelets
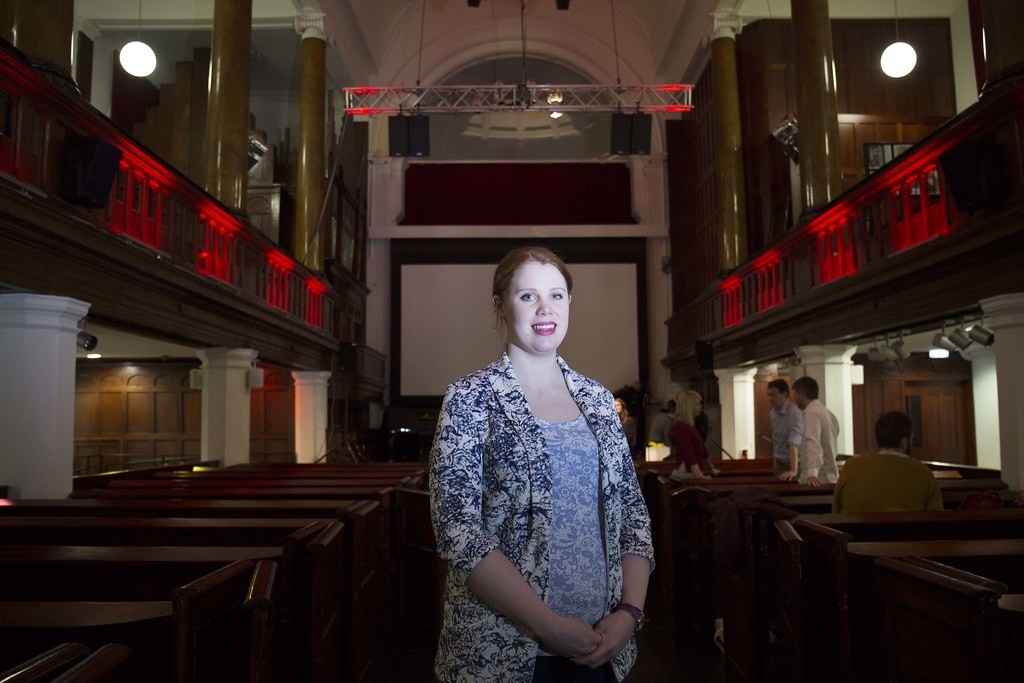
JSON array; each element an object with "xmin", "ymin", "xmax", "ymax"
[{"xmin": 704, "ymin": 463, "xmax": 714, "ymax": 469}]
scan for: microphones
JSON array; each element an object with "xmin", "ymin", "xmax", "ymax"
[
  {"xmin": 708, "ymin": 436, "xmax": 734, "ymax": 460},
  {"xmin": 315, "ymin": 441, "xmax": 346, "ymax": 464}
]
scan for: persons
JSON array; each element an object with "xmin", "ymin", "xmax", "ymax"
[
  {"xmin": 766, "ymin": 376, "xmax": 840, "ymax": 488},
  {"xmin": 832, "ymin": 411, "xmax": 944, "ymax": 513},
  {"xmin": 428, "ymin": 244, "xmax": 656, "ymax": 683},
  {"xmin": 614, "ymin": 391, "xmax": 721, "ymax": 481}
]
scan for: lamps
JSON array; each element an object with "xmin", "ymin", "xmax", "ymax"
[
  {"xmin": 877, "ymin": 1, "xmax": 919, "ymax": 80},
  {"xmin": 77, "ymin": 331, "xmax": 99, "ymax": 352},
  {"xmin": 849, "ymin": 316, "xmax": 997, "ymax": 364},
  {"xmin": 118, "ymin": 0, "xmax": 158, "ymax": 78}
]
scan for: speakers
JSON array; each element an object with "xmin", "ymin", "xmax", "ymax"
[
  {"xmin": 389, "ymin": 114, "xmax": 430, "ymax": 158},
  {"xmin": 610, "ymin": 115, "xmax": 651, "ymax": 156}
]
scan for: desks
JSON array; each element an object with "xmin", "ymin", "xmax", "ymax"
[
  {"xmin": 0, "ymin": 463, "xmax": 432, "ymax": 683},
  {"xmin": 627, "ymin": 455, "xmax": 1024, "ymax": 683}
]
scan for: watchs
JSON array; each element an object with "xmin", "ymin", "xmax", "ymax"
[{"xmin": 611, "ymin": 602, "xmax": 646, "ymax": 633}]
